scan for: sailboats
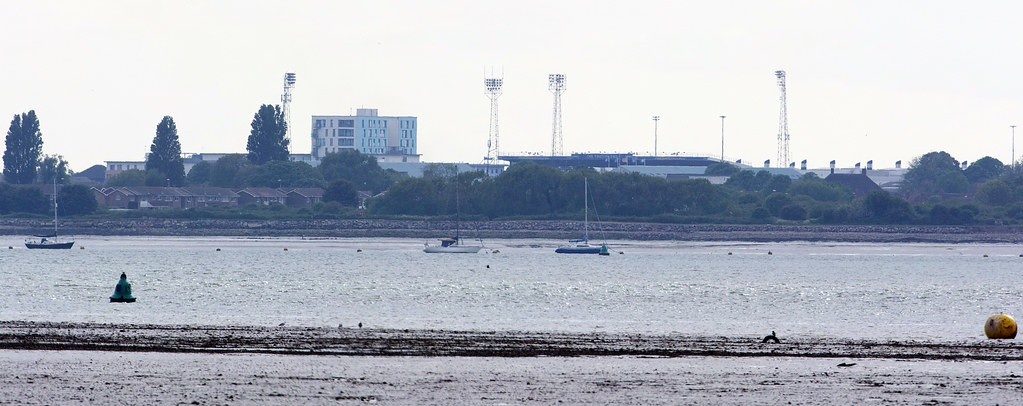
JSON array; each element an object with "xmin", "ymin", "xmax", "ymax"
[
  {"xmin": 422, "ymin": 164, "xmax": 486, "ymax": 254},
  {"xmin": 24, "ymin": 178, "xmax": 75, "ymax": 250},
  {"xmin": 554, "ymin": 177, "xmax": 608, "ymax": 255}
]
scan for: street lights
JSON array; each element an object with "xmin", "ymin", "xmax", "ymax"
[
  {"xmin": 719, "ymin": 115, "xmax": 727, "ymax": 169},
  {"xmin": 1010, "ymin": 126, "xmax": 1016, "ymax": 171},
  {"xmin": 652, "ymin": 115, "xmax": 660, "ymax": 155}
]
